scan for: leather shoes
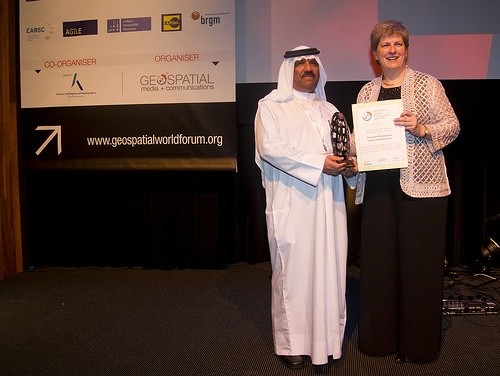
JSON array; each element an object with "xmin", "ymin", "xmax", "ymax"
[{"xmin": 280, "ymin": 355, "xmax": 305, "ymax": 368}]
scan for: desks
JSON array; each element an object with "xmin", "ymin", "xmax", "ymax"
[{"xmin": 21, "ymin": 156, "xmax": 239, "ymax": 269}]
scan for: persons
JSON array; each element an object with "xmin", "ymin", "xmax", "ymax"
[
  {"xmin": 350, "ymin": 19, "xmax": 461, "ymax": 366},
  {"xmin": 252, "ymin": 46, "xmax": 359, "ymax": 369}
]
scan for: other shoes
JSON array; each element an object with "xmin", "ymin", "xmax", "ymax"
[{"xmin": 395, "ymin": 354, "xmax": 408, "ymax": 364}]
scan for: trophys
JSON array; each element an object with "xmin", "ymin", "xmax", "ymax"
[{"xmin": 329, "ymin": 112, "xmax": 357, "ymax": 168}]
